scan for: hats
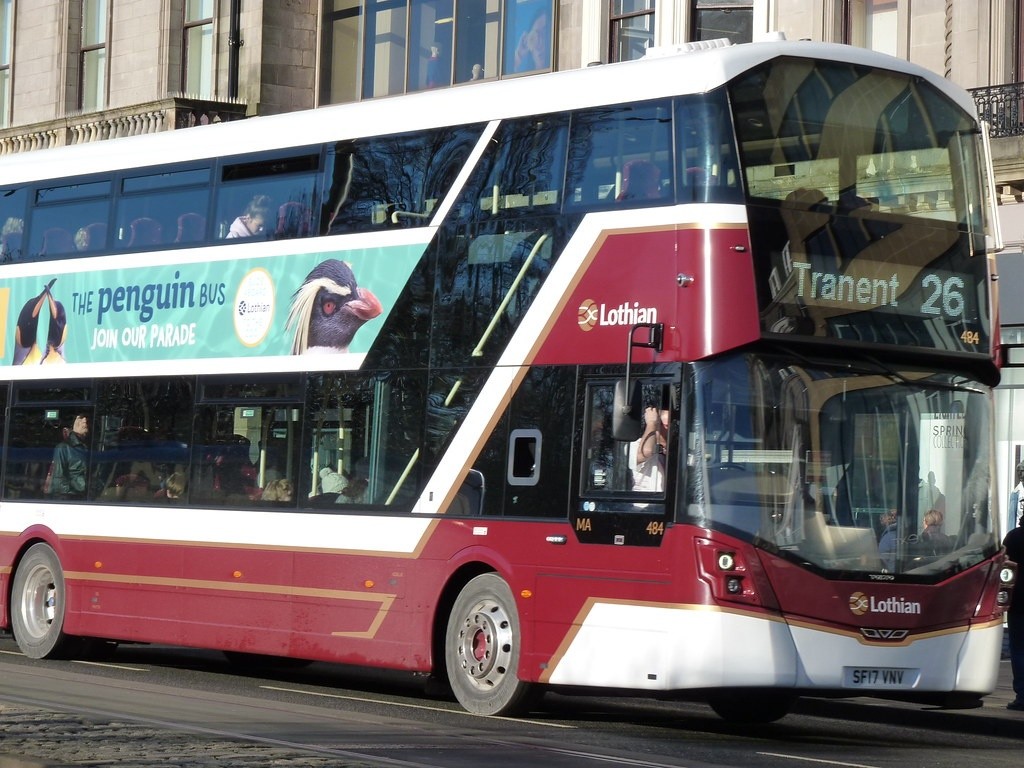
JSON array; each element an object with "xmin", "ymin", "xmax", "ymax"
[{"xmin": 319, "ymin": 467, "xmax": 351, "ymax": 496}]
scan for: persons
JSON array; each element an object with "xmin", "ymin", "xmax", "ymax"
[
  {"xmin": 0, "ymin": 218, "xmax": 89, "ymax": 258},
  {"xmin": 913, "ymin": 511, "xmax": 961, "ymax": 556},
  {"xmin": 22, "ymin": 407, "xmax": 371, "ymax": 503},
  {"xmin": 226, "ymin": 194, "xmax": 274, "ymax": 239},
  {"xmin": 593, "ymin": 403, "xmax": 668, "ymax": 513},
  {"xmin": 878, "ymin": 508, "xmax": 897, "ymax": 573},
  {"xmin": 1002, "ymin": 506, "xmax": 1024, "ymax": 710}
]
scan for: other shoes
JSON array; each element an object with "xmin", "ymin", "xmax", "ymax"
[{"xmin": 1006, "ymin": 698, "xmax": 1024, "ymax": 712}]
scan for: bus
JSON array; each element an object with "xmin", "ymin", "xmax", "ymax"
[{"xmin": 0, "ymin": 29, "xmax": 1024, "ymax": 720}]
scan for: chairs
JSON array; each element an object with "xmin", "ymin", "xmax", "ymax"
[
  {"xmin": 40, "ymin": 229, "xmax": 77, "ymax": 254},
  {"xmin": 680, "ymin": 169, "xmax": 712, "ymax": 187},
  {"xmin": 172, "ymin": 212, "xmax": 207, "ymax": 244},
  {"xmin": 126, "ymin": 218, "xmax": 163, "ymax": 248},
  {"xmin": 274, "ymin": 201, "xmax": 311, "ymax": 238},
  {"xmin": 2, "ymin": 232, "xmax": 24, "ymax": 260},
  {"xmin": 616, "ymin": 158, "xmax": 660, "ymax": 203},
  {"xmin": 82, "ymin": 224, "xmax": 108, "ymax": 252}
]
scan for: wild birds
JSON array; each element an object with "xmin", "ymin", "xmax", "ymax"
[{"xmin": 284, "ymin": 258, "xmax": 382, "ymax": 353}]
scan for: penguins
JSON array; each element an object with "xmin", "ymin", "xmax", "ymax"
[{"xmin": 11, "ymin": 278, "xmax": 66, "ymax": 366}]
